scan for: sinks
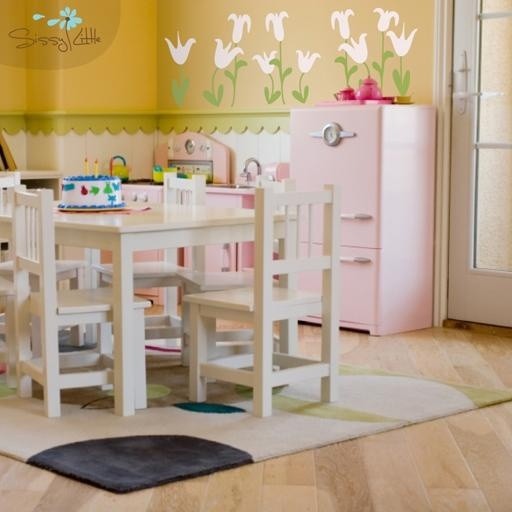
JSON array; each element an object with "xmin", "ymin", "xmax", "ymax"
[{"xmin": 208, "ymin": 184, "xmax": 253, "ymax": 190}]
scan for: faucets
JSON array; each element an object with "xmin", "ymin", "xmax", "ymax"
[{"xmin": 240, "ymin": 157, "xmax": 262, "ymax": 179}]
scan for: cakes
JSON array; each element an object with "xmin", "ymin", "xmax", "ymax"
[{"xmin": 61, "ymin": 174, "xmax": 122, "ymax": 208}]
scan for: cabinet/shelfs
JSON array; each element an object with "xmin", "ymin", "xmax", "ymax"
[{"xmin": 286, "ymin": 104, "xmax": 435, "ymax": 339}]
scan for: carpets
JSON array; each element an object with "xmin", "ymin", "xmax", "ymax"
[{"xmin": 0, "ymin": 363, "xmax": 512, "ymax": 495}]
